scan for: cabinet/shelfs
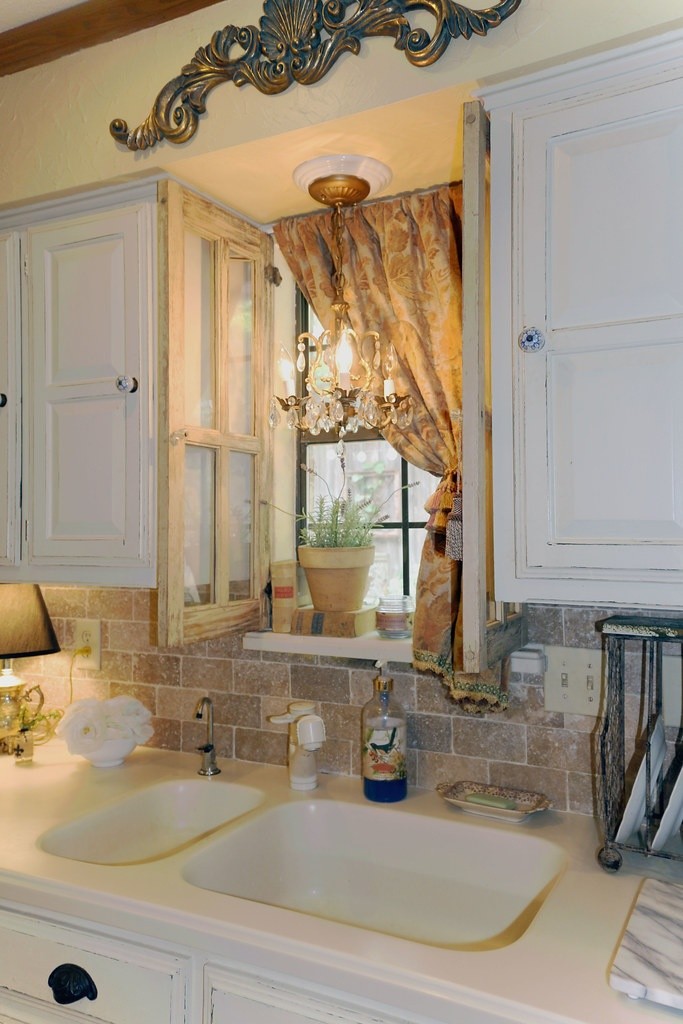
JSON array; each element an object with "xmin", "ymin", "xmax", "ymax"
[
  {"xmin": 472, "ymin": 26, "xmax": 683, "ymax": 614},
  {"xmin": 2, "ymin": 173, "xmax": 159, "ymax": 592},
  {"xmin": 2, "ymin": 879, "xmax": 455, "ymax": 1022}
]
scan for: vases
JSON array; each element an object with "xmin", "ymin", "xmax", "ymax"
[
  {"xmin": 78, "ymin": 734, "xmax": 137, "ymax": 768},
  {"xmin": 296, "ymin": 543, "xmax": 376, "ymax": 615},
  {"xmin": 14, "ymin": 732, "xmax": 35, "ymax": 767}
]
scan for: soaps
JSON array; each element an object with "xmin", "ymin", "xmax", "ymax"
[{"xmin": 467, "ymin": 793, "xmax": 517, "ymax": 811}]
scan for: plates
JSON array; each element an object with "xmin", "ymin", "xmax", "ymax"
[
  {"xmin": 649, "ymin": 765, "xmax": 682, "ymax": 855},
  {"xmin": 613, "ymin": 716, "xmax": 670, "ymax": 844},
  {"xmin": 438, "ymin": 777, "xmax": 550, "ymax": 824}
]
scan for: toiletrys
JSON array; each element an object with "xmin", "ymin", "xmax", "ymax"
[
  {"xmin": 194, "ymin": 698, "xmax": 221, "ymax": 775},
  {"xmin": 359, "ymin": 660, "xmax": 408, "ymax": 804}
]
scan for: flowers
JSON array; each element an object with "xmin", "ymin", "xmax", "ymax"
[
  {"xmin": 16, "ymin": 702, "xmax": 62, "ymax": 742},
  {"xmin": 53, "ymin": 694, "xmax": 159, "ymax": 758},
  {"xmin": 255, "ymin": 456, "xmax": 421, "ymax": 548}
]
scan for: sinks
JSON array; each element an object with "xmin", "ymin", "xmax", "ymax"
[
  {"xmin": 35, "ymin": 772, "xmax": 270, "ymax": 869},
  {"xmin": 176, "ymin": 797, "xmax": 570, "ymax": 952}
]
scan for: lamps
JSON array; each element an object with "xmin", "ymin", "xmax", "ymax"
[
  {"xmin": 1, "ymin": 582, "xmax": 62, "ymax": 755},
  {"xmin": 264, "ymin": 154, "xmax": 417, "ymax": 453}
]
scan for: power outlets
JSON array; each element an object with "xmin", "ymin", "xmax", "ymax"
[{"xmin": 72, "ymin": 616, "xmax": 102, "ymax": 671}]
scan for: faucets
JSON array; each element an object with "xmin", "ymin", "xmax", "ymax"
[{"xmin": 270, "ymin": 701, "xmax": 326, "ymax": 791}]
